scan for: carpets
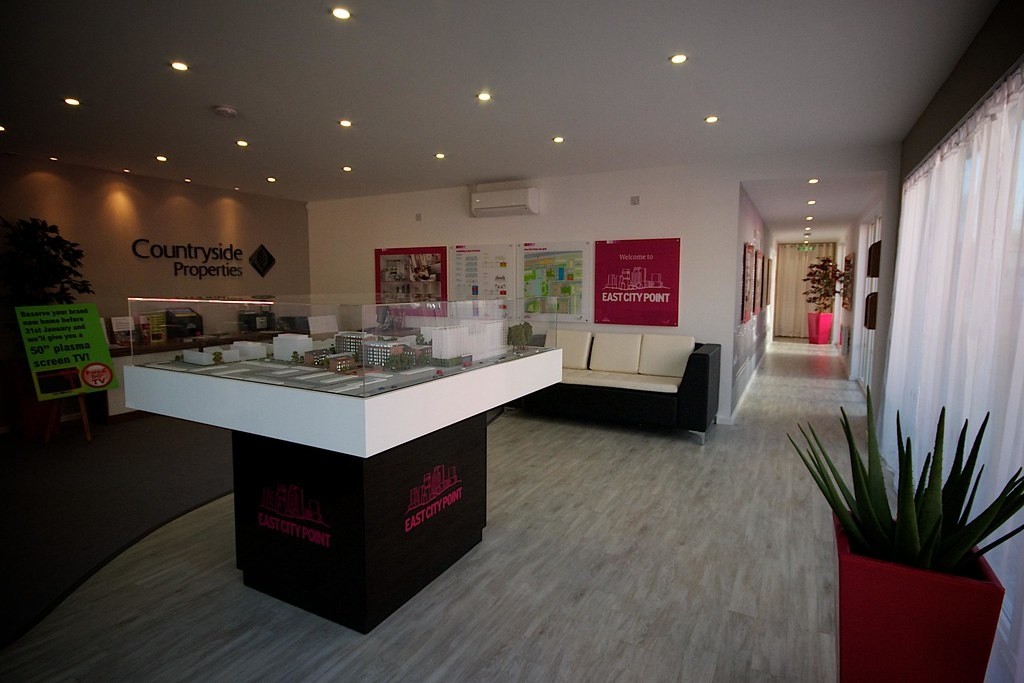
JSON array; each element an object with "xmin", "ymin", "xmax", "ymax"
[{"xmin": 0, "ymin": 398, "xmax": 503, "ymax": 656}]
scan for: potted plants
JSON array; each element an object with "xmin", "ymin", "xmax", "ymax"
[
  {"xmin": 786, "ymin": 385, "xmax": 1024, "ymax": 683},
  {"xmin": 802, "ymin": 256, "xmax": 847, "ymax": 344}
]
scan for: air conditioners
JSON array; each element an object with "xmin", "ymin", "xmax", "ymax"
[{"xmin": 470, "ymin": 188, "xmax": 540, "ymax": 217}]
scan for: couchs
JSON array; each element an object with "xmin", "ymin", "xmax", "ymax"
[{"xmin": 502, "ymin": 329, "xmax": 721, "ymax": 444}]
